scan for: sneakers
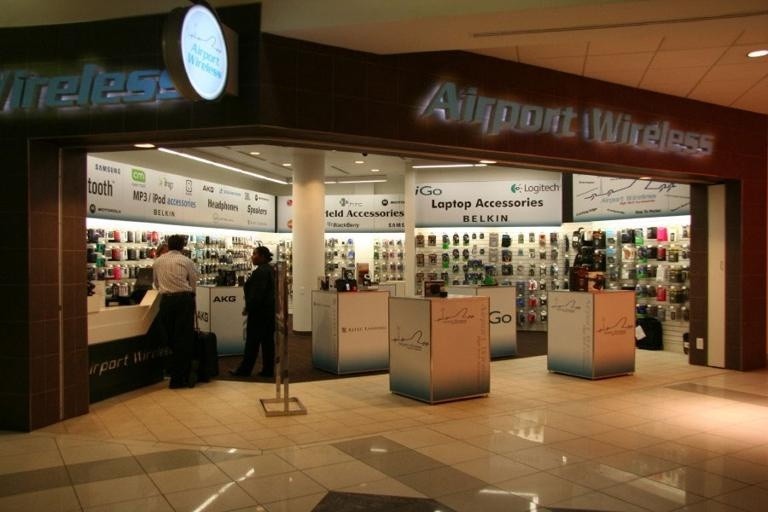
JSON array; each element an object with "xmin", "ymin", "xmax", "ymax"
[
  {"xmin": 168, "ymin": 373, "xmax": 198, "ymax": 388},
  {"xmin": 228, "ymin": 366, "xmax": 248, "ymax": 377}
]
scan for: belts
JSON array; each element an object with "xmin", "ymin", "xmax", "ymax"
[{"xmin": 161, "ymin": 291, "xmax": 196, "ymax": 297}]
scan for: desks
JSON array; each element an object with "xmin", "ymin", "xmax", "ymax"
[
  {"xmin": 308, "ymin": 286, "xmax": 388, "ymax": 373},
  {"xmin": 385, "ymin": 294, "xmax": 493, "ymax": 404},
  {"xmin": 194, "ymin": 283, "xmax": 255, "ymax": 357},
  {"xmin": 545, "ymin": 287, "xmax": 636, "ymax": 380},
  {"xmin": 445, "ymin": 282, "xmax": 518, "ymax": 359},
  {"xmin": 359, "ymin": 282, "xmax": 396, "ymax": 297}
]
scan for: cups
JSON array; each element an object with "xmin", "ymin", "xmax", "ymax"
[
  {"xmin": 439, "ymin": 291, "xmax": 447, "ymax": 297},
  {"xmin": 113, "ymin": 286, "xmax": 129, "ymax": 296}
]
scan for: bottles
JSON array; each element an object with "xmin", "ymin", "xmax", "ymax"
[{"xmin": 572, "ymin": 231, "xmax": 580, "ymax": 247}]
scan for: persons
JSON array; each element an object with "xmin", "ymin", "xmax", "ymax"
[
  {"xmin": 228, "ymin": 245, "xmax": 276, "ymax": 376},
  {"xmin": 153, "ymin": 235, "xmax": 197, "ymax": 388}
]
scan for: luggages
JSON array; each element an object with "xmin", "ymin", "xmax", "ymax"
[{"xmin": 194, "ymin": 328, "xmax": 219, "ymax": 383}]
